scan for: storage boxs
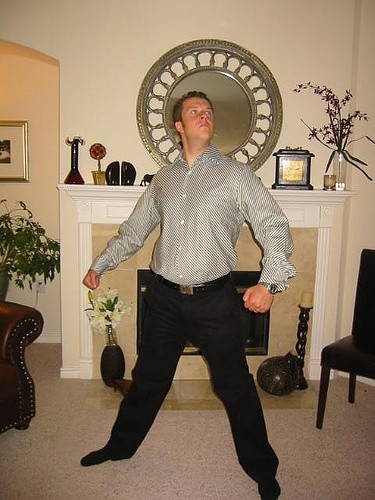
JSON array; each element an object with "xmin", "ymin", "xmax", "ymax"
[{"xmin": 271, "ymin": 146, "xmax": 318, "ymax": 190}]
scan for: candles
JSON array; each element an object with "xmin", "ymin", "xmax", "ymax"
[
  {"xmin": 299, "ymin": 287, "xmax": 314, "ymax": 306},
  {"xmin": 325, "ymin": 177, "xmax": 335, "ymax": 189}
]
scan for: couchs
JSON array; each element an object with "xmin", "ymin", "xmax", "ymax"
[{"xmin": 0, "ymin": 300, "xmax": 46, "ymax": 435}]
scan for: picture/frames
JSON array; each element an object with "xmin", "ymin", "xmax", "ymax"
[{"xmin": 0, "ymin": 120, "xmax": 31, "ymax": 182}]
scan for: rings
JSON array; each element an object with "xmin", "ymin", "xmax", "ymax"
[{"xmin": 255, "ymin": 310, "xmax": 258, "ymax": 313}]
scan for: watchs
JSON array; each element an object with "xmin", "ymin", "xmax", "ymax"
[{"xmin": 258, "ymin": 282, "xmax": 278, "ymax": 295}]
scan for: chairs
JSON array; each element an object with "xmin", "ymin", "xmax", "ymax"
[{"xmin": 314, "ymin": 248, "xmax": 375, "ymax": 431}]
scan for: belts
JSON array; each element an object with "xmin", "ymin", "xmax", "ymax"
[{"xmin": 149, "ymin": 269, "xmax": 231, "ymax": 295}]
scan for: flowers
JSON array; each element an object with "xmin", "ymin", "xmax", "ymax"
[
  {"xmin": 76, "ymin": 288, "xmax": 131, "ymax": 346},
  {"xmin": 289, "ymin": 77, "xmax": 375, "ymax": 182},
  {"xmin": 0, "ymin": 199, "xmax": 60, "ymax": 293}
]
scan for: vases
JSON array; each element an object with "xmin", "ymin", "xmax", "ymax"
[
  {"xmin": 63, "ymin": 142, "xmax": 85, "ymax": 184},
  {"xmin": 0, "ymin": 261, "xmax": 12, "ymax": 303},
  {"xmin": 333, "ymin": 152, "xmax": 348, "ymax": 190},
  {"xmin": 98, "ymin": 343, "xmax": 126, "ymax": 389}
]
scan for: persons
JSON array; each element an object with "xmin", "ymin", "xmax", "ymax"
[{"xmin": 80, "ymin": 91, "xmax": 297, "ymax": 500}]
